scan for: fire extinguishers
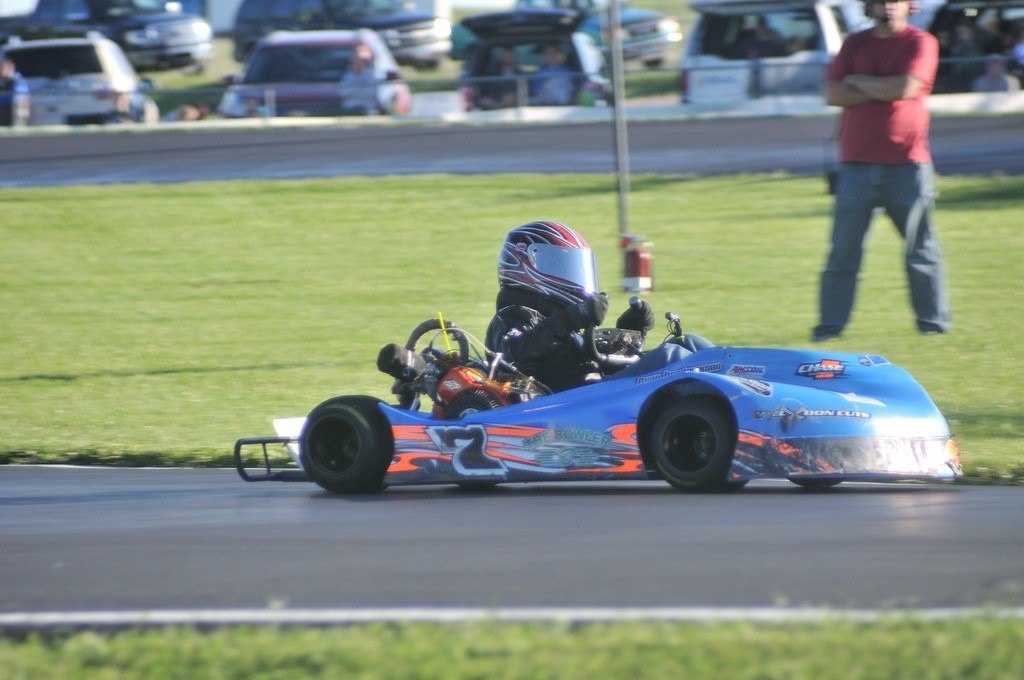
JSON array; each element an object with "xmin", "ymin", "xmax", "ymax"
[{"xmin": 619, "ymin": 233, "xmax": 653, "ymax": 293}]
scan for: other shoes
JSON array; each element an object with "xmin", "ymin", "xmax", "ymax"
[
  {"xmin": 919, "ymin": 322, "xmax": 943, "ymax": 334},
  {"xmin": 816, "ymin": 325, "xmax": 841, "ymax": 339}
]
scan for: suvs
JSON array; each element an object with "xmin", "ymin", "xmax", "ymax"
[
  {"xmin": 231, "ymin": 0, "xmax": 452, "ymax": 70},
  {"xmin": 677, "ymin": 0, "xmax": 875, "ymax": 107},
  {"xmin": 3, "ymin": 31, "xmax": 159, "ymax": 126},
  {"xmin": 457, "ymin": 10, "xmax": 613, "ymax": 111}
]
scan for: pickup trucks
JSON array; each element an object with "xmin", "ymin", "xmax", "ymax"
[{"xmin": 0, "ymin": 0, "xmax": 211, "ymax": 77}]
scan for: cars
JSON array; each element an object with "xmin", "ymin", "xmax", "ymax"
[
  {"xmin": 451, "ymin": 0, "xmax": 682, "ymax": 71},
  {"xmin": 219, "ymin": 25, "xmax": 413, "ymax": 117},
  {"xmin": 917, "ymin": 0, "xmax": 1024, "ymax": 92}
]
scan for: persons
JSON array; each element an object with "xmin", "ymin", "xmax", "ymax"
[
  {"xmin": 731, "ymin": 14, "xmax": 804, "ymax": 59},
  {"xmin": 109, "ymin": 91, "xmax": 273, "ymax": 125},
  {"xmin": 481, "ymin": 41, "xmax": 575, "ymax": 106},
  {"xmin": 810, "ymin": 0, "xmax": 952, "ymax": 341},
  {"xmin": 932, "ymin": 9, "xmax": 1024, "ymax": 93},
  {"xmin": 484, "ymin": 221, "xmax": 717, "ymax": 393},
  {"xmin": 337, "ymin": 57, "xmax": 377, "ymax": 115},
  {"xmin": 0, "ymin": 58, "xmax": 31, "ymax": 127}
]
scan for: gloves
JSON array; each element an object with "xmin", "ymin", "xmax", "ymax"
[
  {"xmin": 616, "ymin": 301, "xmax": 656, "ymax": 339},
  {"xmin": 566, "ymin": 292, "xmax": 610, "ymax": 330}
]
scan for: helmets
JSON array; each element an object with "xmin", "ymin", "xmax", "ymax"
[{"xmin": 497, "ymin": 220, "xmax": 596, "ymax": 307}]
